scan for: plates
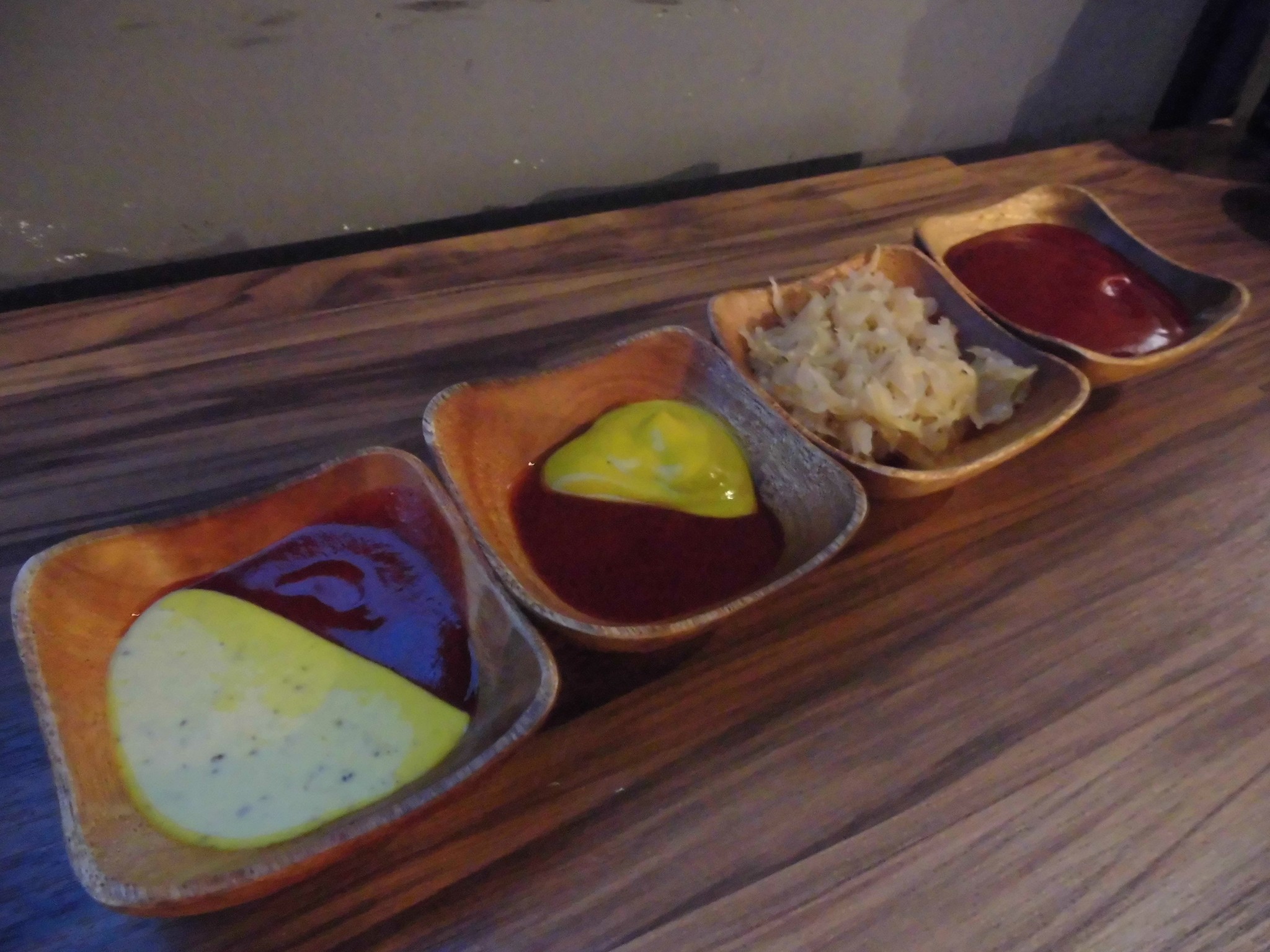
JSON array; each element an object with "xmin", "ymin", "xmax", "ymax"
[
  {"xmin": 707, "ymin": 243, "xmax": 1090, "ymax": 498},
  {"xmin": 916, "ymin": 184, "xmax": 1250, "ymax": 387},
  {"xmin": 421, "ymin": 321, "xmax": 868, "ymax": 652},
  {"xmin": 8, "ymin": 447, "xmax": 559, "ymax": 919}
]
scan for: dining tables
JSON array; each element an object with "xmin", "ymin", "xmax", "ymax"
[{"xmin": 1, "ymin": 144, "xmax": 1270, "ymax": 951}]
